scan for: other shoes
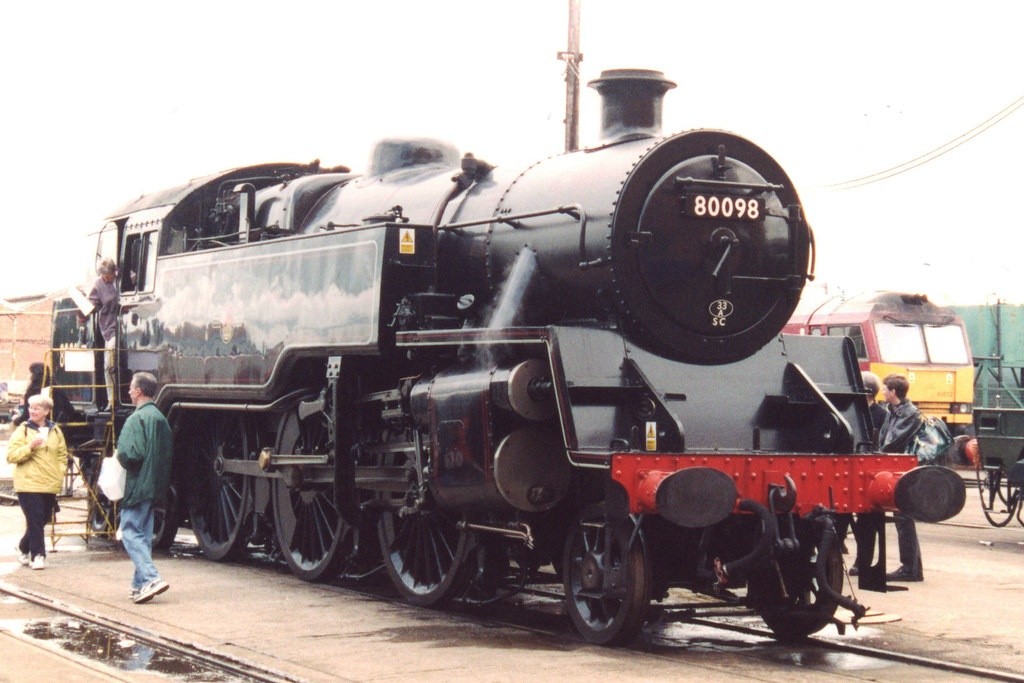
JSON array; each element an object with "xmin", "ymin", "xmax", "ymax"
[
  {"xmin": 849, "ymin": 563, "xmax": 861, "ymax": 575},
  {"xmin": 105, "ymin": 405, "xmax": 117, "ymax": 411},
  {"xmin": 884, "ymin": 566, "xmax": 924, "ymax": 582}
]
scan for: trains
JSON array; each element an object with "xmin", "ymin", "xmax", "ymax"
[
  {"xmin": 0, "ymin": 68, "xmax": 968, "ymax": 648},
  {"xmin": 779, "ymin": 292, "xmax": 975, "ymax": 432}
]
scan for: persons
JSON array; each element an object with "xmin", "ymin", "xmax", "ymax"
[
  {"xmin": 842, "ymin": 372, "xmax": 887, "ymax": 577},
  {"xmin": 78, "ymin": 259, "xmax": 119, "ymax": 411},
  {"xmin": 6, "ymin": 394, "xmax": 69, "ymax": 570},
  {"xmin": 10, "ymin": 363, "xmax": 56, "ymax": 523},
  {"xmin": 877, "ymin": 374, "xmax": 925, "ymax": 582},
  {"xmin": 117, "ymin": 371, "xmax": 169, "ymax": 604}
]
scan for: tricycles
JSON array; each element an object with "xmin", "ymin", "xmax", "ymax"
[{"xmin": 976, "ymin": 408, "xmax": 1024, "ymax": 528}]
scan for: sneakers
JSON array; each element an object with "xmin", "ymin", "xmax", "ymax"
[
  {"xmin": 129, "ymin": 577, "xmax": 169, "ymax": 603},
  {"xmin": 15, "ymin": 543, "xmax": 30, "ymax": 565},
  {"xmin": 31, "ymin": 554, "xmax": 45, "ymax": 570}
]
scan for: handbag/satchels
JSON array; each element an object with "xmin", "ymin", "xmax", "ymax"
[
  {"xmin": 97, "ymin": 449, "xmax": 127, "ymax": 503},
  {"xmin": 905, "ymin": 410, "xmax": 955, "ymax": 466}
]
